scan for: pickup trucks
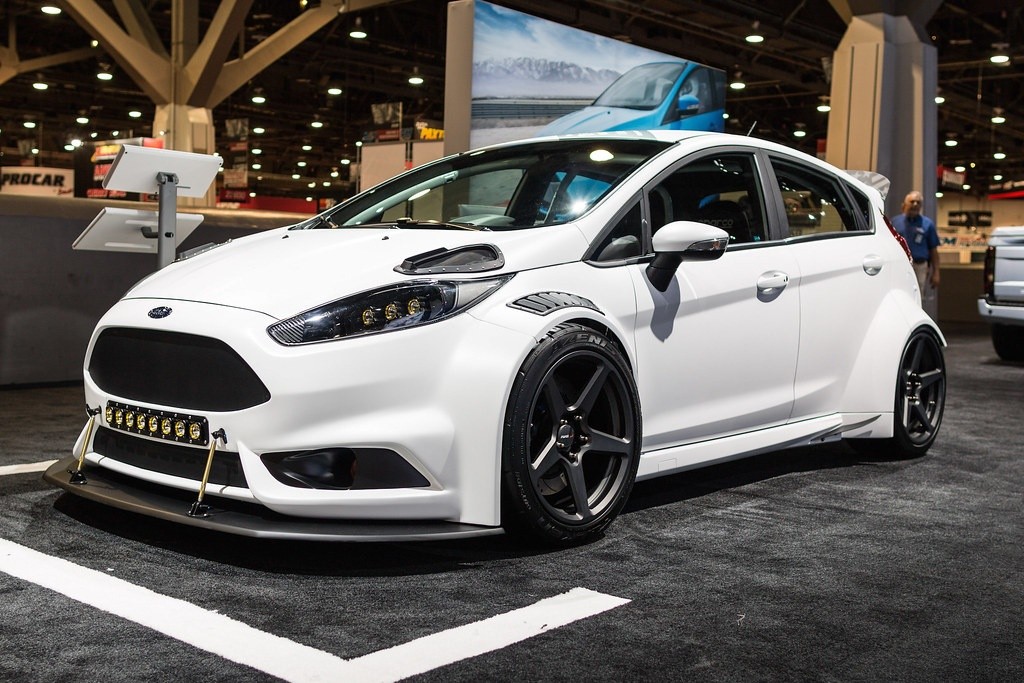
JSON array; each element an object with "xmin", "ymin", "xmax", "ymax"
[{"xmin": 976, "ymin": 223, "xmax": 1024, "ymax": 365}]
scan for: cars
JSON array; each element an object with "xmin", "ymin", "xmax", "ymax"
[
  {"xmin": 40, "ymin": 127, "xmax": 949, "ymax": 545},
  {"xmin": 532, "ymin": 61, "xmax": 728, "ymax": 138}
]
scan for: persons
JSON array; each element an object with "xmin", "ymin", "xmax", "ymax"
[{"xmin": 889, "ymin": 190, "xmax": 940, "ymax": 298}]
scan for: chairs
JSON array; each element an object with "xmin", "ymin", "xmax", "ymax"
[{"xmin": 606, "ymin": 193, "xmax": 753, "ymax": 247}]
